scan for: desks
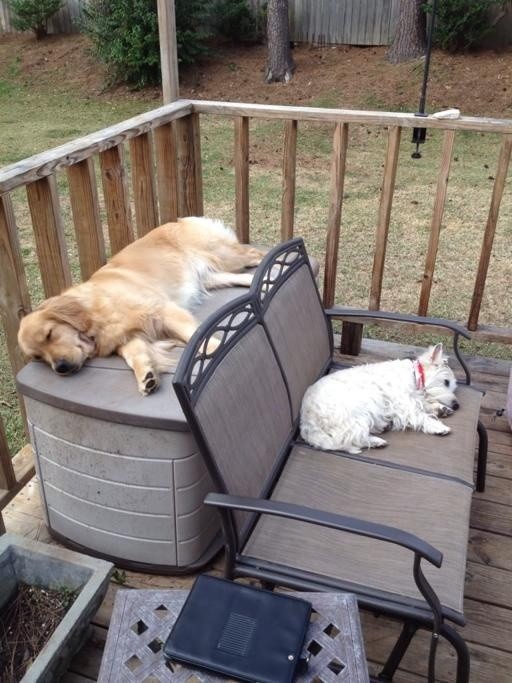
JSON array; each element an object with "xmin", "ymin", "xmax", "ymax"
[{"xmin": 104, "ymin": 582, "xmax": 370, "ymax": 683}]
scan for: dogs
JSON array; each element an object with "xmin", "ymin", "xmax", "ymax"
[
  {"xmin": 298, "ymin": 342, "xmax": 460, "ymax": 456},
  {"xmin": 16, "ymin": 216, "xmax": 276, "ymax": 397}
]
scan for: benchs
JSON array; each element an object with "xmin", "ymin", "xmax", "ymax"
[{"xmin": 172, "ymin": 234, "xmax": 491, "ymax": 683}]
